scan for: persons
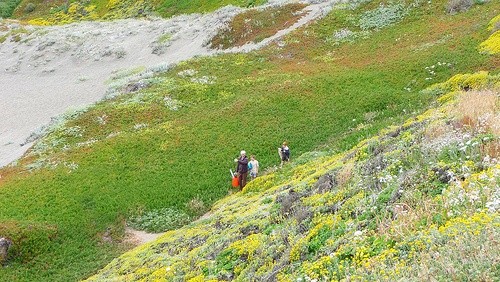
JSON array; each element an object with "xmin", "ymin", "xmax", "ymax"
[
  {"xmin": 248, "ymin": 155, "xmax": 259, "ymax": 181},
  {"xmin": 234, "ymin": 151, "xmax": 248, "ymax": 191},
  {"xmin": 278, "ymin": 141, "xmax": 290, "ymax": 168}
]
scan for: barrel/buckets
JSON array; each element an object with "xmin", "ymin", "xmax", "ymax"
[{"xmin": 232, "ymin": 176, "xmax": 239, "ymax": 187}]
